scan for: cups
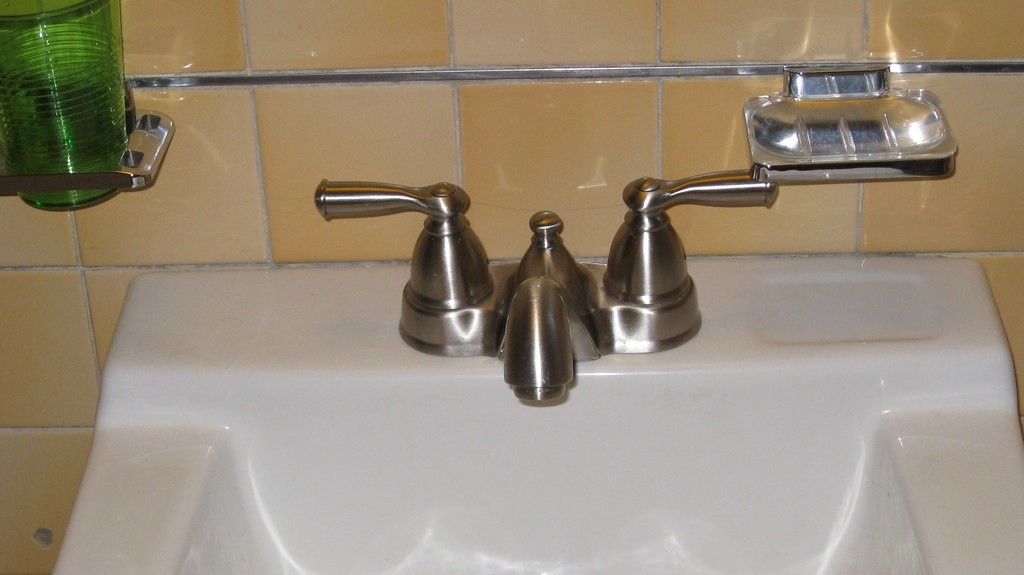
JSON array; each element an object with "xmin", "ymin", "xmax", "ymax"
[{"xmin": 0, "ymin": 0, "xmax": 129, "ymax": 213}]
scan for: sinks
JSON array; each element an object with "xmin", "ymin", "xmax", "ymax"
[{"xmin": 51, "ymin": 252, "xmax": 1023, "ymax": 575}]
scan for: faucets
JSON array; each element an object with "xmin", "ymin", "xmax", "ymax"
[{"xmin": 497, "ymin": 209, "xmax": 600, "ymax": 407}]
